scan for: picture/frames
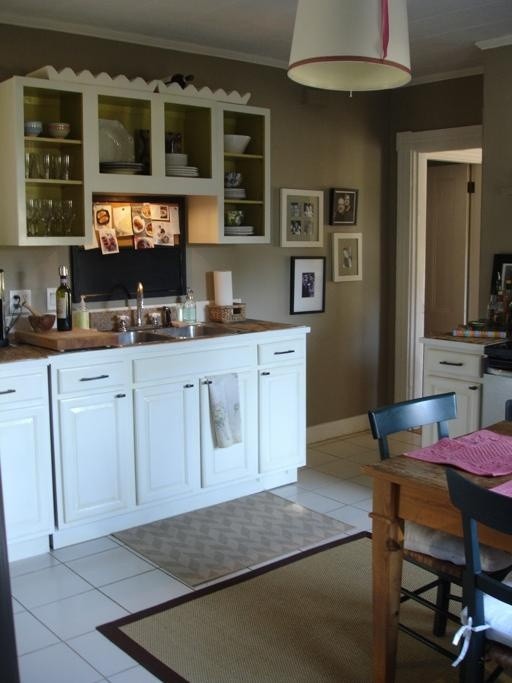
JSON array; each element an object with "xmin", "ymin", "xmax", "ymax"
[
  {"xmin": 290, "ymin": 256, "xmax": 325, "ymax": 314},
  {"xmin": 280, "ymin": 188, "xmax": 323, "ymax": 248},
  {"xmin": 491, "ymin": 252, "xmax": 512, "ymax": 300},
  {"xmin": 331, "ymin": 232, "xmax": 363, "ymax": 283},
  {"xmin": 329, "ymin": 188, "xmax": 358, "ymax": 225}
]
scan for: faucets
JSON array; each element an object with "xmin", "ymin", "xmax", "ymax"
[
  {"xmin": 136, "ymin": 282, "xmax": 144, "ymax": 324},
  {"xmin": 164, "ymin": 306, "xmax": 172, "ymax": 326}
]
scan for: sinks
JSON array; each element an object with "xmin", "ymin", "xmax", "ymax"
[
  {"xmin": 119, "ymin": 331, "xmax": 177, "ymax": 347},
  {"xmin": 152, "ymin": 324, "xmax": 243, "ymax": 337}
]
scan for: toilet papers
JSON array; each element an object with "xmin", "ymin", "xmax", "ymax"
[{"xmin": 214, "ymin": 270, "xmax": 233, "ymax": 306}]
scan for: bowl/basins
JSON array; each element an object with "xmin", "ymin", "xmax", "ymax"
[
  {"xmin": 29, "ymin": 313, "xmax": 55, "ymax": 331},
  {"xmin": 224, "ymin": 170, "xmax": 243, "ymax": 187},
  {"xmin": 223, "ymin": 131, "xmax": 251, "ymax": 152},
  {"xmin": 466, "ymin": 319, "xmax": 485, "ymax": 329},
  {"xmin": 226, "ymin": 208, "xmax": 247, "ymax": 225}
]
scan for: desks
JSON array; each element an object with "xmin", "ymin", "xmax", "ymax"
[{"xmin": 358, "ymin": 416, "xmax": 512, "ymax": 682}]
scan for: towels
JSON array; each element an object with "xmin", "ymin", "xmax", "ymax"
[
  {"xmin": 486, "ymin": 479, "xmax": 512, "ymax": 497},
  {"xmin": 208, "ymin": 374, "xmax": 242, "ymax": 448},
  {"xmin": 403, "ymin": 430, "xmax": 511, "ymax": 477}
]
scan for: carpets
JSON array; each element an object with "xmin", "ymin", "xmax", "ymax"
[{"xmin": 96, "ymin": 531, "xmax": 512, "ymax": 683}]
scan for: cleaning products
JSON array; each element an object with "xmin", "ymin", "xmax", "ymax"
[
  {"xmin": 76, "ymin": 295, "xmax": 90, "ymax": 330},
  {"xmin": 185, "ymin": 288, "xmax": 197, "ymax": 323}
]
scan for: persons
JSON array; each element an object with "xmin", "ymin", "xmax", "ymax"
[
  {"xmin": 343, "ymin": 195, "xmax": 352, "ymax": 221},
  {"xmin": 334, "ymin": 197, "xmax": 349, "ymax": 222}
]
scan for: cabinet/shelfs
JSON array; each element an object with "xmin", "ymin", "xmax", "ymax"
[
  {"xmin": 418, "ymin": 337, "xmax": 485, "ymax": 449},
  {"xmin": 187, "ymin": 101, "xmax": 271, "ymax": 245},
  {"xmin": 0, "ymin": 75, "xmax": 93, "ymax": 247},
  {"xmin": 0, "ymin": 358, "xmax": 54, "ymax": 564},
  {"xmin": 93, "ymin": 84, "xmax": 217, "ymax": 196},
  {"xmin": 132, "ymin": 333, "xmax": 258, "ymax": 534},
  {"xmin": 47, "ymin": 350, "xmax": 135, "ymax": 549},
  {"xmin": 256, "ymin": 325, "xmax": 312, "ymax": 494}
]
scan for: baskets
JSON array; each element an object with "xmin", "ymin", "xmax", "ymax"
[{"xmin": 206, "ymin": 305, "xmax": 245, "ymax": 323}]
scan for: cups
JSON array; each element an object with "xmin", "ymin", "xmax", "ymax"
[{"xmin": 25, "ymin": 118, "xmax": 72, "ymax": 138}]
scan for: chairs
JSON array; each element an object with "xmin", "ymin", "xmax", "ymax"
[
  {"xmin": 443, "ymin": 465, "xmax": 512, "ymax": 682},
  {"xmin": 369, "ymin": 392, "xmax": 512, "ymax": 635}
]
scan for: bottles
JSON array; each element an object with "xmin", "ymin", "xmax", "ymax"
[
  {"xmin": 486, "ymin": 268, "xmax": 512, "ymax": 327},
  {"xmin": 172, "ymin": 294, "xmax": 184, "ymax": 320},
  {"xmin": 0, "ymin": 269, "xmax": 6, "ymax": 340},
  {"xmin": 55, "ymin": 265, "xmax": 73, "ymax": 331},
  {"xmin": 184, "ymin": 286, "xmax": 198, "ymax": 323}
]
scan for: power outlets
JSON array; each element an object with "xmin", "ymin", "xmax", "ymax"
[{"xmin": 9, "ymin": 290, "xmax": 31, "ymax": 314}]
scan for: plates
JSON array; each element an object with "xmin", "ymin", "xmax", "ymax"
[
  {"xmin": 165, "ymin": 151, "xmax": 200, "ymax": 179},
  {"xmin": 94, "ymin": 202, "xmax": 169, "ymax": 252},
  {"xmin": 223, "ymin": 225, "xmax": 256, "ymax": 235},
  {"xmin": 220, "ymin": 188, "xmax": 249, "ymax": 198},
  {"xmin": 98, "ymin": 158, "xmax": 143, "ymax": 174},
  {"xmin": 99, "ymin": 117, "xmax": 136, "ymax": 160}
]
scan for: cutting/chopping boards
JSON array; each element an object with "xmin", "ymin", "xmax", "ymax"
[{"xmin": 15, "ymin": 327, "xmax": 122, "ymax": 353}]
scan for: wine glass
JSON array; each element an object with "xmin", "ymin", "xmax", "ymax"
[{"xmin": 24, "ymin": 151, "xmax": 82, "ymax": 237}]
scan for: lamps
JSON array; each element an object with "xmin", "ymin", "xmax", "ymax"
[{"xmin": 285, "ymin": 0, "xmax": 413, "ymax": 95}]
